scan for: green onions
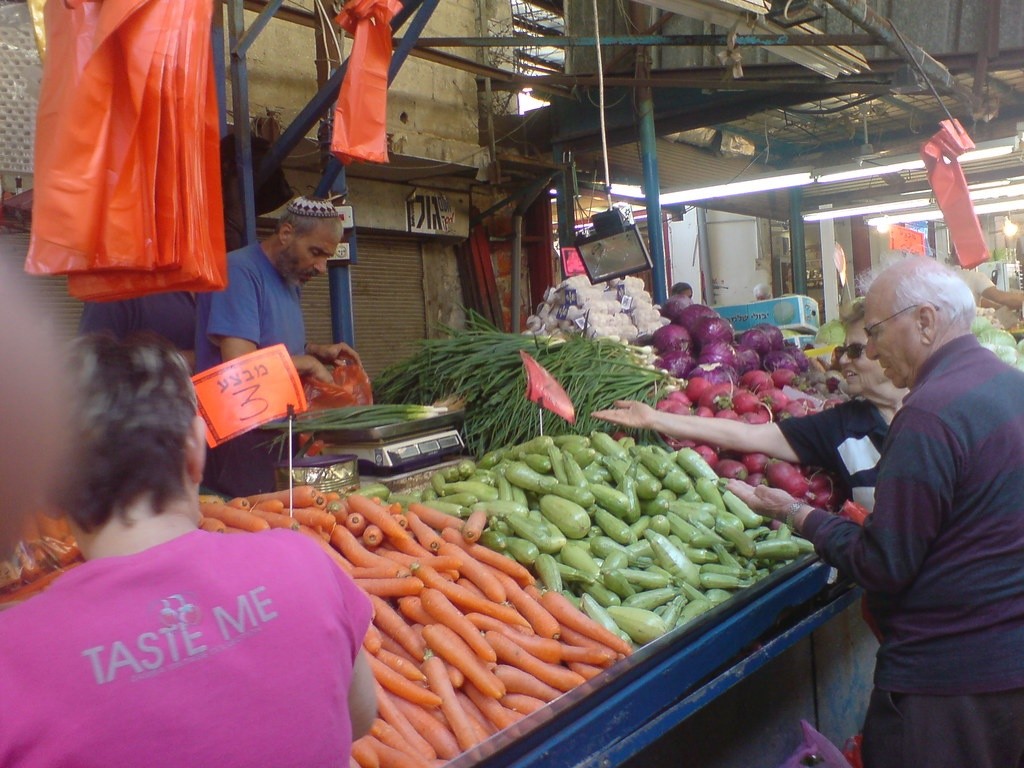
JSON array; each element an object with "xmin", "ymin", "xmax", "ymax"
[
  {"xmin": 370, "ymin": 300, "xmax": 687, "ymax": 456},
  {"xmin": 252, "ymin": 394, "xmax": 467, "ymax": 462}
]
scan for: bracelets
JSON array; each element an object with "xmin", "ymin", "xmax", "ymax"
[{"xmin": 787, "ymin": 500, "xmax": 803, "ymax": 538}]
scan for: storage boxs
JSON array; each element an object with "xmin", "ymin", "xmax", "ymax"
[
  {"xmin": 804, "ymin": 345, "xmax": 837, "ymax": 366},
  {"xmin": 711, "ymin": 295, "xmax": 820, "ymax": 335},
  {"xmin": 783, "ymin": 334, "xmax": 816, "ymax": 349}
]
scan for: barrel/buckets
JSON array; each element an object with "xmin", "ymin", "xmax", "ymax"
[{"xmin": 275, "ymin": 454, "xmax": 359, "ymax": 493}]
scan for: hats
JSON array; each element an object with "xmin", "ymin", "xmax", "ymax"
[{"xmin": 286, "ymin": 195, "xmax": 339, "ymax": 217}]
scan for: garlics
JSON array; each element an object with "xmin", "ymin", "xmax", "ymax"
[{"xmin": 519, "ymin": 275, "xmax": 666, "ymax": 342}]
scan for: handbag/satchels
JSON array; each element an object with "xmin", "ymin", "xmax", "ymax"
[{"xmin": 298, "ymin": 364, "xmax": 374, "ymax": 457}]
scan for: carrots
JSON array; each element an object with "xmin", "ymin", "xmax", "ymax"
[{"xmin": 0, "ymin": 487, "xmax": 633, "ymax": 768}]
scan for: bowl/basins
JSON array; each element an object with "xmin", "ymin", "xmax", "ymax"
[{"xmin": 988, "ymin": 248, "xmax": 1016, "ymax": 262}]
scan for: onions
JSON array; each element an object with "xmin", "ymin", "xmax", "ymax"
[{"xmin": 629, "ymin": 293, "xmax": 808, "ymax": 385}]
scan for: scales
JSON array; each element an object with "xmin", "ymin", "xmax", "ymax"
[{"xmin": 309, "ymin": 406, "xmax": 467, "ymax": 474}]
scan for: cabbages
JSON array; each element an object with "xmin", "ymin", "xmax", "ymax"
[
  {"xmin": 781, "ymin": 329, "xmax": 801, "ymax": 340},
  {"xmin": 970, "ymin": 314, "xmax": 1024, "ymax": 373},
  {"xmin": 815, "ymin": 320, "xmax": 845, "ymax": 344}
]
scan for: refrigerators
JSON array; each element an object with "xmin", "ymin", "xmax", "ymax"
[{"xmin": 978, "ymin": 263, "xmax": 1020, "ymax": 314}]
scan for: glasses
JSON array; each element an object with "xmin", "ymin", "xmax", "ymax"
[
  {"xmin": 835, "ymin": 342, "xmax": 867, "ymax": 360},
  {"xmin": 863, "ymin": 305, "xmax": 938, "ymax": 336}
]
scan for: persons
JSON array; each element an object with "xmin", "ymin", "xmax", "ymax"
[
  {"xmin": 589, "ymin": 299, "xmax": 911, "ymax": 641},
  {"xmin": 197, "ymin": 197, "xmax": 363, "ymax": 499},
  {"xmin": 724, "ymin": 257, "xmax": 1024, "ymax": 768},
  {"xmin": 950, "ymin": 243, "xmax": 1024, "ymax": 311},
  {"xmin": 672, "ymin": 282, "xmax": 693, "ymax": 300},
  {"xmin": 0, "ymin": 331, "xmax": 378, "ymax": 768},
  {"xmin": 754, "ymin": 283, "xmax": 771, "ymax": 301},
  {"xmin": 81, "ymin": 288, "xmax": 199, "ymax": 378}
]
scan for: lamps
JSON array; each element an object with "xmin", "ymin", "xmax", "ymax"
[
  {"xmin": 810, "ymin": 135, "xmax": 1020, "ymax": 183},
  {"xmin": 572, "ymin": 0, "xmax": 653, "ymax": 285},
  {"xmin": 659, "ymin": 165, "xmax": 817, "ymax": 205}
]
